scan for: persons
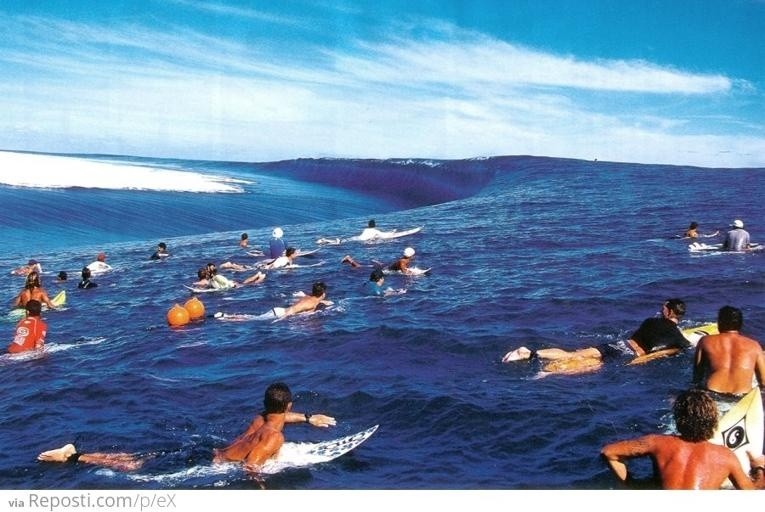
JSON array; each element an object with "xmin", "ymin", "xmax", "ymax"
[
  {"xmin": 693, "ymin": 306, "xmax": 765, "ymax": 402},
  {"xmin": 0, "ymin": 252, "xmax": 113, "ymax": 356},
  {"xmin": 193, "ymin": 219, "xmax": 415, "ymax": 324},
  {"xmin": 501, "ymin": 299, "xmax": 693, "ymax": 375},
  {"xmin": 723, "ymin": 219, "xmax": 750, "ymax": 251},
  {"xmin": 600, "ymin": 389, "xmax": 765, "ymax": 489},
  {"xmin": 36, "ymin": 382, "xmax": 337, "ymax": 476},
  {"xmin": 150, "ymin": 243, "xmax": 173, "ymax": 260},
  {"xmin": 685, "ymin": 222, "xmax": 698, "ymax": 238}
]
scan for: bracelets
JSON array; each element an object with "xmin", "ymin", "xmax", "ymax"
[{"xmin": 752, "ymin": 465, "xmax": 765, "ymax": 479}]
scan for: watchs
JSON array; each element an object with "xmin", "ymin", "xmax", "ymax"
[{"xmin": 305, "ymin": 412, "xmax": 312, "ymax": 423}]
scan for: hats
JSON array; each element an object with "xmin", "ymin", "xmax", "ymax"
[
  {"xmin": 272, "ymin": 228, "xmax": 283, "ymax": 238},
  {"xmin": 729, "ymin": 220, "xmax": 743, "ymax": 228},
  {"xmin": 404, "ymin": 248, "xmax": 415, "ymax": 257}
]
